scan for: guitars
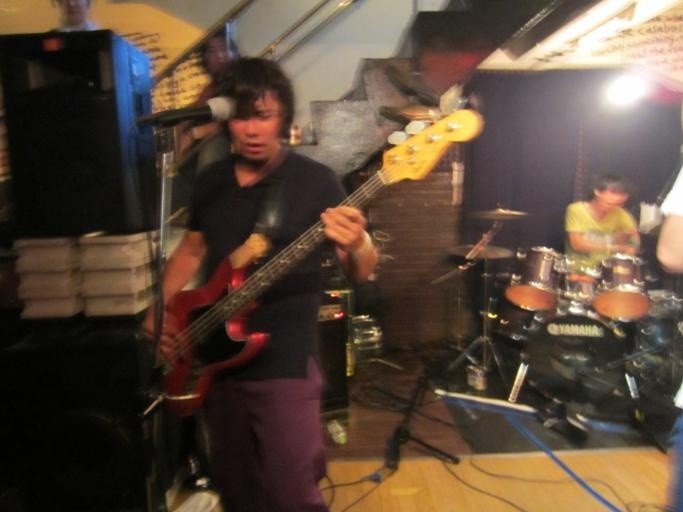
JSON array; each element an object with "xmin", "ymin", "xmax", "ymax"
[{"xmin": 137, "ymin": 97, "xmax": 483, "ymax": 412}]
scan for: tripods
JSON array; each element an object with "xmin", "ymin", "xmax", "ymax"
[{"xmin": 446, "ymin": 272, "xmax": 512, "ymax": 392}]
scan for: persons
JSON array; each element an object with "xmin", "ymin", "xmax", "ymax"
[
  {"xmin": 548, "ymin": 170, "xmax": 641, "ymax": 415},
  {"xmin": 171, "ymin": 26, "xmax": 240, "ymax": 164},
  {"xmin": 655, "ymin": 165, "xmax": 682, "ymax": 511},
  {"xmin": 138, "ymin": 55, "xmax": 380, "ymax": 511},
  {"xmin": 48, "ymin": 1, "xmax": 101, "ymax": 32}
]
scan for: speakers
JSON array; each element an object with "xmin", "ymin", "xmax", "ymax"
[
  {"xmin": 0, "ymin": 28, "xmax": 162, "ymax": 234},
  {"xmin": 315, "ymin": 271, "xmax": 349, "ymax": 423}
]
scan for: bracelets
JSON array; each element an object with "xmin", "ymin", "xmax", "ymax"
[
  {"xmin": 628, "ymin": 238, "xmax": 639, "ymax": 248},
  {"xmin": 350, "ymin": 231, "xmax": 372, "ymax": 261},
  {"xmin": 606, "ymin": 242, "xmax": 614, "ymax": 255}
]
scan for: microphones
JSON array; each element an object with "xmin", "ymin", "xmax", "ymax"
[{"xmin": 136, "ymin": 96, "xmax": 233, "ymax": 134}]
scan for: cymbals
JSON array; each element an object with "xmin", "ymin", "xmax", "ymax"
[
  {"xmin": 472, "ymin": 209, "xmax": 528, "ymax": 221},
  {"xmin": 449, "ymin": 244, "xmax": 514, "ymax": 259}
]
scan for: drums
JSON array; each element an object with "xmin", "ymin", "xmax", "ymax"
[
  {"xmin": 353, "ymin": 325, "xmax": 382, "ymax": 377},
  {"xmin": 495, "ymin": 247, "xmax": 650, "ymax": 342},
  {"xmin": 627, "ymin": 288, "xmax": 682, "ymax": 397},
  {"xmin": 522, "ymin": 303, "xmax": 628, "ymax": 405}
]
